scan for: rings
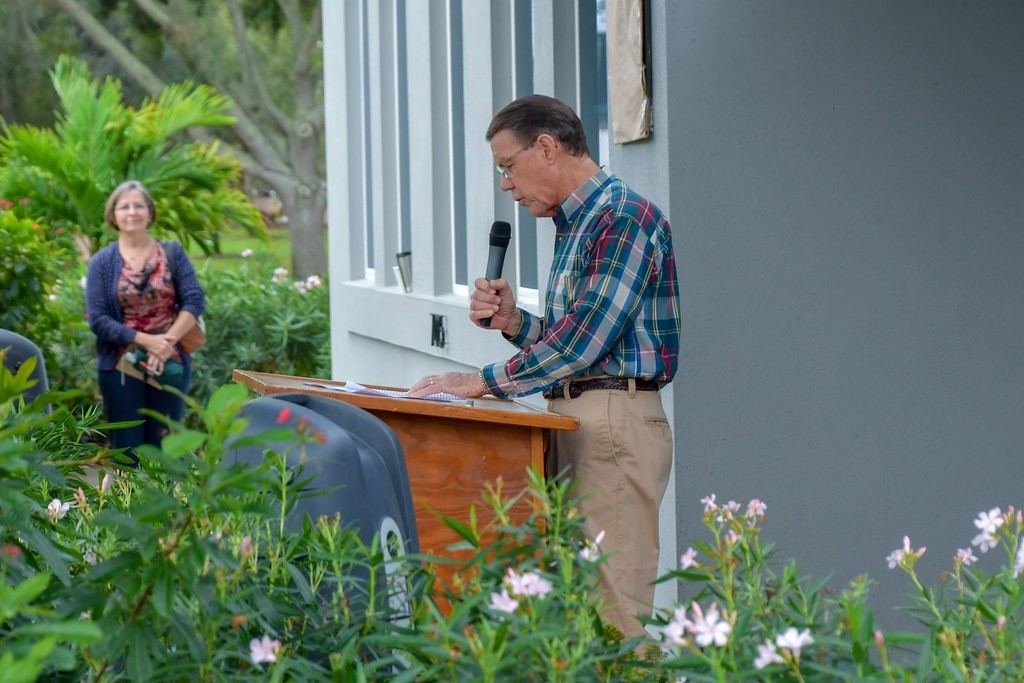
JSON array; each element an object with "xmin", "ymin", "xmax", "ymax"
[{"xmin": 430, "ymin": 381, "xmax": 433, "ymax": 384}]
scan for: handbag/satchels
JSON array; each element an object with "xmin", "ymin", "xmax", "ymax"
[{"xmin": 169, "ymin": 240, "xmax": 206, "ymax": 351}]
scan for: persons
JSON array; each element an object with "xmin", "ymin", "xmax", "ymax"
[
  {"xmin": 85, "ymin": 180, "xmax": 206, "ymax": 468},
  {"xmin": 404, "ymin": 94, "xmax": 682, "ymax": 683}
]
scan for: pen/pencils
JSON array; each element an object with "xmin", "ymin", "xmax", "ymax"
[{"xmin": 140, "ymin": 360, "xmax": 160, "ymax": 376}]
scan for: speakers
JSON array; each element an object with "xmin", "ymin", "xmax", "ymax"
[
  {"xmin": 0, "ymin": 328, "xmax": 51, "ymax": 416},
  {"xmin": 211, "ymin": 393, "xmax": 421, "ymax": 678}
]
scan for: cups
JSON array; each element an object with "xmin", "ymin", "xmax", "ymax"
[
  {"xmin": 397, "ymin": 252, "xmax": 412, "ymax": 293},
  {"xmin": 365, "ymin": 268, "xmax": 375, "ymax": 285},
  {"xmin": 393, "ymin": 267, "xmax": 403, "ymax": 289}
]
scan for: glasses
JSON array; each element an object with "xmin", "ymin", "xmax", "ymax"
[{"xmin": 495, "ymin": 140, "xmax": 557, "ymax": 179}]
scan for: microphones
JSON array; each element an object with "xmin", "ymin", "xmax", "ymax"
[{"xmin": 480, "ymin": 221, "xmax": 510, "ymax": 326}]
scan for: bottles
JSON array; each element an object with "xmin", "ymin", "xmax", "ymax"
[{"xmin": 125, "ymin": 352, "xmax": 164, "ymax": 379}]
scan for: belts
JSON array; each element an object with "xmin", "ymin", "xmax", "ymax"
[{"xmin": 543, "ymin": 378, "xmax": 659, "ymax": 399}]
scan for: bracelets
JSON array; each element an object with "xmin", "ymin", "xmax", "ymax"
[{"xmin": 479, "ymin": 371, "xmax": 490, "ymax": 395}]
